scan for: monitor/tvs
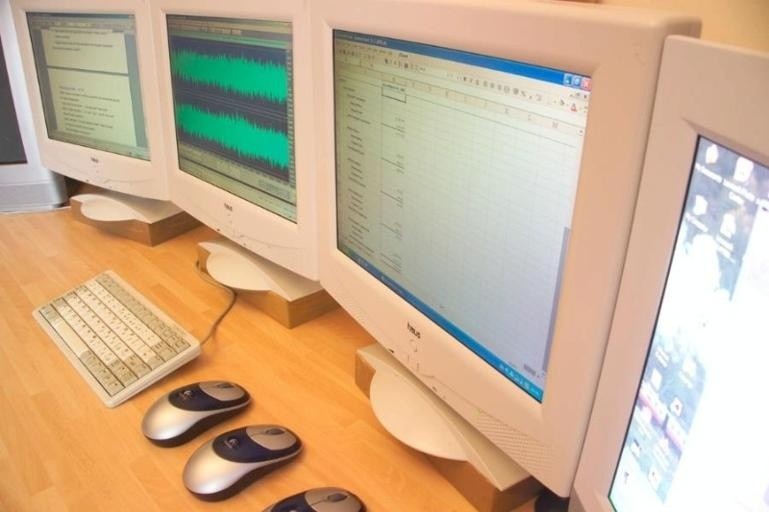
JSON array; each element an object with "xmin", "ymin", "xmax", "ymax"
[
  {"xmin": 11, "ymin": 0, "xmax": 183, "ymax": 227},
  {"xmin": 567, "ymin": 35, "xmax": 769, "ymax": 512},
  {"xmin": 311, "ymin": 0, "xmax": 708, "ymax": 505},
  {"xmin": 0, "ymin": 0, "xmax": 70, "ymax": 214},
  {"xmin": 152, "ymin": 0, "xmax": 313, "ymax": 308}
]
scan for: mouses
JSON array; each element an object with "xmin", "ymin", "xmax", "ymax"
[
  {"xmin": 262, "ymin": 486, "xmax": 367, "ymax": 512},
  {"xmin": 180, "ymin": 422, "xmax": 304, "ymax": 500},
  {"xmin": 141, "ymin": 379, "xmax": 254, "ymax": 448}
]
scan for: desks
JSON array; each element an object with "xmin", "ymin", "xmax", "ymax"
[{"xmin": 1, "ymin": 206, "xmax": 540, "ymax": 512}]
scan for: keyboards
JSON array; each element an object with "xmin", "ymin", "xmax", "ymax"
[{"xmin": 30, "ymin": 268, "xmax": 205, "ymax": 408}]
